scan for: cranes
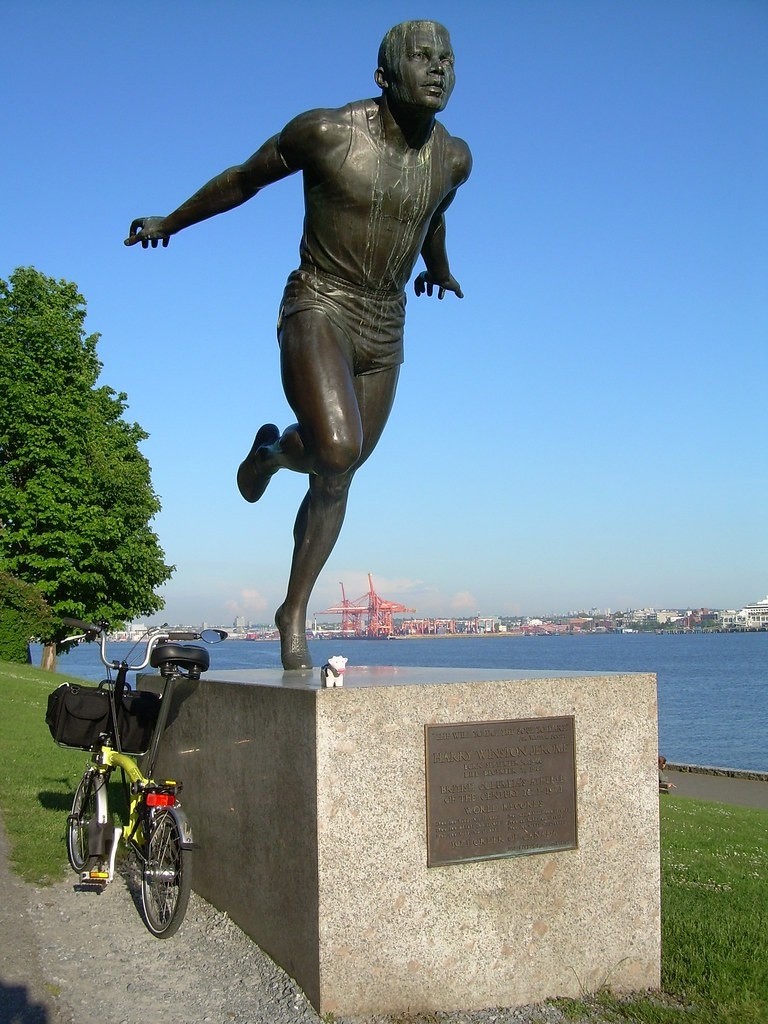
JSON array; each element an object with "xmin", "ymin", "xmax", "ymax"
[{"xmin": 314, "ymin": 573, "xmax": 416, "ymax": 641}]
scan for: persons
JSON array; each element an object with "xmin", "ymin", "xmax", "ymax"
[
  {"xmin": 124, "ymin": 18, "xmax": 472, "ymax": 671},
  {"xmin": 659, "ymin": 756, "xmax": 676, "ymax": 789}
]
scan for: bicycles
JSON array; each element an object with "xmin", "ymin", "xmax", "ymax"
[{"xmin": 48, "ymin": 616, "xmax": 229, "ymax": 940}]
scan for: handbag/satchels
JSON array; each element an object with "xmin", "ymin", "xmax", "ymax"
[{"xmin": 46, "ymin": 678, "xmax": 160, "ymax": 755}]
